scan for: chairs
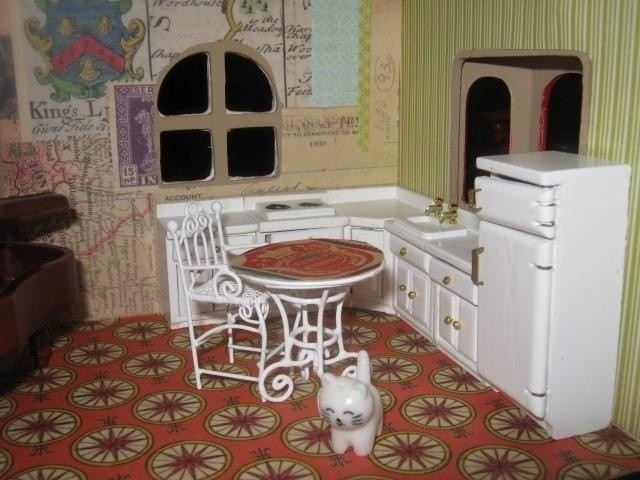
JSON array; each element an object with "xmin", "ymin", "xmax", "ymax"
[{"xmin": 167, "ymin": 199, "xmax": 293, "ymax": 402}]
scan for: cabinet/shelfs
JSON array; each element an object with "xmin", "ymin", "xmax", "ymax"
[
  {"xmin": 429, "ymin": 250, "xmax": 477, "ymax": 379},
  {"xmin": 152, "ymin": 199, "xmax": 258, "ymax": 329},
  {"xmin": 389, "ymin": 230, "xmax": 430, "ymax": 341},
  {"xmin": 347, "ymin": 226, "xmax": 392, "ymax": 314},
  {"xmin": 475, "ymin": 150, "xmax": 634, "ymax": 441}
]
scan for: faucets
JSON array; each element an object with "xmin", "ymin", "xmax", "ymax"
[
  {"xmin": 438, "ymin": 202, "xmax": 460, "ymax": 225},
  {"xmin": 424, "ymin": 196, "xmax": 445, "ymax": 219}
]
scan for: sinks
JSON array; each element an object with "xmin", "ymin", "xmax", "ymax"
[{"xmin": 393, "ymin": 211, "xmax": 468, "ymax": 240}]
